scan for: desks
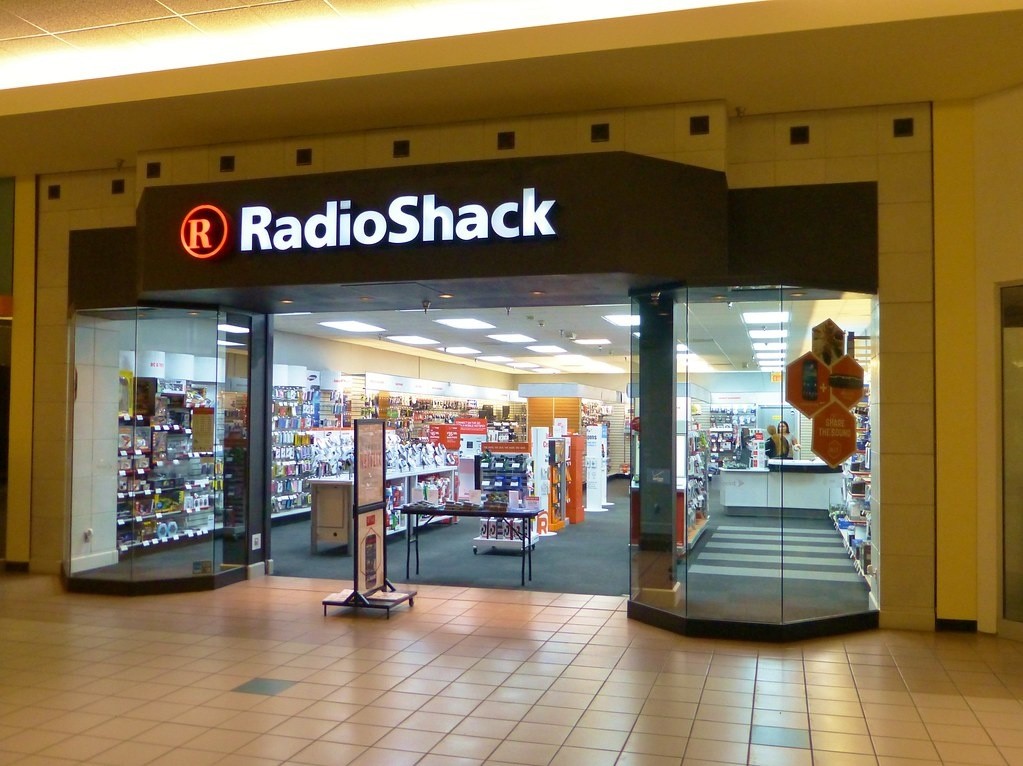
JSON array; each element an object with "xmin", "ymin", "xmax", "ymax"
[{"xmin": 395, "ymin": 502, "xmax": 545, "ymax": 587}]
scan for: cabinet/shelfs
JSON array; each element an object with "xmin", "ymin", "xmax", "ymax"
[
  {"xmin": 830, "ymin": 382, "xmax": 871, "ymax": 579},
  {"xmin": 118, "ymin": 379, "xmax": 455, "ymax": 559}
]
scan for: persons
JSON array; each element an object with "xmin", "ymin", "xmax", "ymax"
[
  {"xmin": 778, "ymin": 421, "xmax": 799, "ymax": 461},
  {"xmin": 765, "ymin": 425, "xmax": 790, "ymax": 459}
]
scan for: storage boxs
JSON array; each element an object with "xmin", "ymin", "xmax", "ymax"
[{"xmin": 837, "ymin": 399, "xmax": 871, "ymax": 573}]
